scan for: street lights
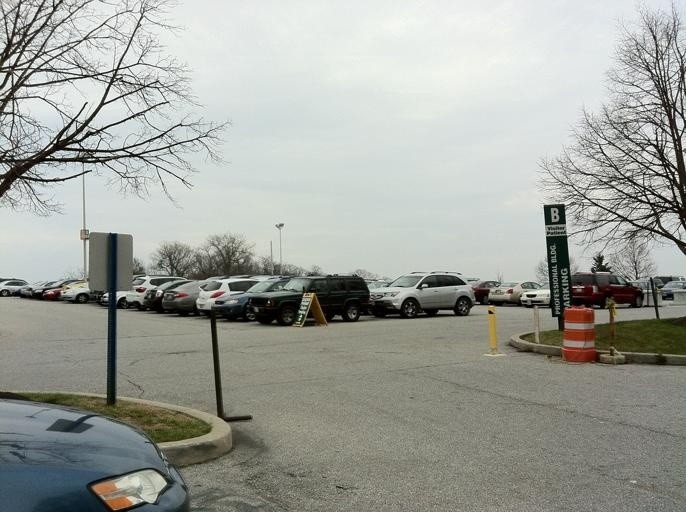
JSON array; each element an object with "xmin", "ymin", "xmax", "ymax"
[
  {"xmin": 79, "ymin": 150, "xmax": 95, "ymax": 280},
  {"xmin": 275, "ymin": 222, "xmax": 284, "ymax": 274}
]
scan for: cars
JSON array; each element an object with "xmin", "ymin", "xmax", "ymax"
[
  {"xmin": 365, "ymin": 278, "xmax": 389, "ymax": 289},
  {"xmin": 0, "ymin": 273, "xmax": 298, "ymax": 316},
  {"xmin": 0, "ymin": 397, "xmax": 191, "ymax": 509},
  {"xmin": 469, "ymin": 278, "xmax": 551, "ymax": 310},
  {"xmin": 626, "ymin": 277, "xmax": 686, "ymax": 292}
]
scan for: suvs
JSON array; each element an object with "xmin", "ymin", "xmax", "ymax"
[
  {"xmin": 572, "ymin": 272, "xmax": 644, "ymax": 308},
  {"xmin": 247, "ymin": 274, "xmax": 369, "ymax": 326},
  {"xmin": 368, "ymin": 271, "xmax": 476, "ymax": 318}
]
